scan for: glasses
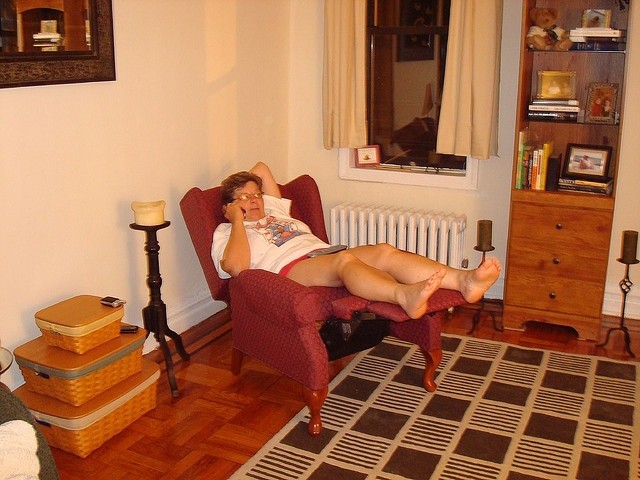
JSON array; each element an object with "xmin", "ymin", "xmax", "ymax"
[{"xmin": 232, "ymin": 191, "xmax": 265, "ymax": 202}]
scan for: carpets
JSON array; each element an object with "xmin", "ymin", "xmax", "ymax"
[{"xmin": 224, "ymin": 329, "xmax": 639, "ymax": 479}]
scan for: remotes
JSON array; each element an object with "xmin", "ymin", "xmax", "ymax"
[{"xmin": 306, "ymin": 244, "xmax": 349, "ymax": 258}]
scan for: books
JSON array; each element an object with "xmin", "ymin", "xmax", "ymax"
[
  {"xmin": 525, "ymin": 95, "xmax": 581, "ymax": 122},
  {"xmin": 514, "ymin": 125, "xmax": 563, "ymax": 194},
  {"xmin": 568, "ymin": 25, "xmax": 627, "ymax": 51},
  {"xmin": 555, "ymin": 174, "xmax": 614, "ymax": 198}
]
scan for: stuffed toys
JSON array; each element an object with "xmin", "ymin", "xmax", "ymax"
[{"xmin": 525, "ymin": 6, "xmax": 574, "ymax": 51}]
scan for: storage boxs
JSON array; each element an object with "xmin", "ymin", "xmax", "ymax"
[
  {"xmin": 10, "ymin": 355, "xmax": 161, "ymax": 459},
  {"xmin": 13, "ymin": 322, "xmax": 151, "ymax": 407},
  {"xmin": 32, "ymin": 294, "xmax": 126, "ymax": 353}
]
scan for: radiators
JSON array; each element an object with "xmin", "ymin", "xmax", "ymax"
[{"xmin": 323, "ymin": 198, "xmax": 467, "ymax": 272}]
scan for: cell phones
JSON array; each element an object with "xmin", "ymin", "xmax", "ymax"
[
  {"xmin": 222, "ymin": 201, "xmax": 246, "ymax": 220},
  {"xmin": 119, "ymin": 325, "xmax": 139, "ymax": 334},
  {"xmin": 99, "ymin": 296, "xmax": 127, "ymax": 308}
]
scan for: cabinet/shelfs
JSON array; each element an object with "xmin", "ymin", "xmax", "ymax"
[{"xmin": 501, "ymin": 1, "xmax": 631, "ymax": 343}]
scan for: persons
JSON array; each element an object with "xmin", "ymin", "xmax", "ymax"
[{"xmin": 209, "ymin": 161, "xmax": 503, "ymax": 322}]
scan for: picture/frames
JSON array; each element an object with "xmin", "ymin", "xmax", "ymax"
[
  {"xmin": 354, "ymin": 145, "xmax": 382, "ymax": 168},
  {"xmin": 584, "ymin": 82, "xmax": 620, "ymax": 127},
  {"xmin": 581, "ymin": 8, "xmax": 612, "ymax": 29},
  {"xmin": 561, "ymin": 143, "xmax": 613, "ymax": 184},
  {"xmin": 0, "ymin": 0, "xmax": 117, "ymax": 88},
  {"xmin": 536, "ymin": 70, "xmax": 576, "ymax": 100}
]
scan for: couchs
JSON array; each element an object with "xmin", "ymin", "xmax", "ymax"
[{"xmin": 180, "ymin": 176, "xmax": 470, "ymax": 438}]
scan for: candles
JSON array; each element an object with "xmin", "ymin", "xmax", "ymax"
[
  {"xmin": 133, "ymin": 197, "xmax": 166, "ymax": 225},
  {"xmin": 619, "ymin": 226, "xmax": 638, "ymax": 262},
  {"xmin": 476, "ymin": 216, "xmax": 494, "ymax": 249}
]
